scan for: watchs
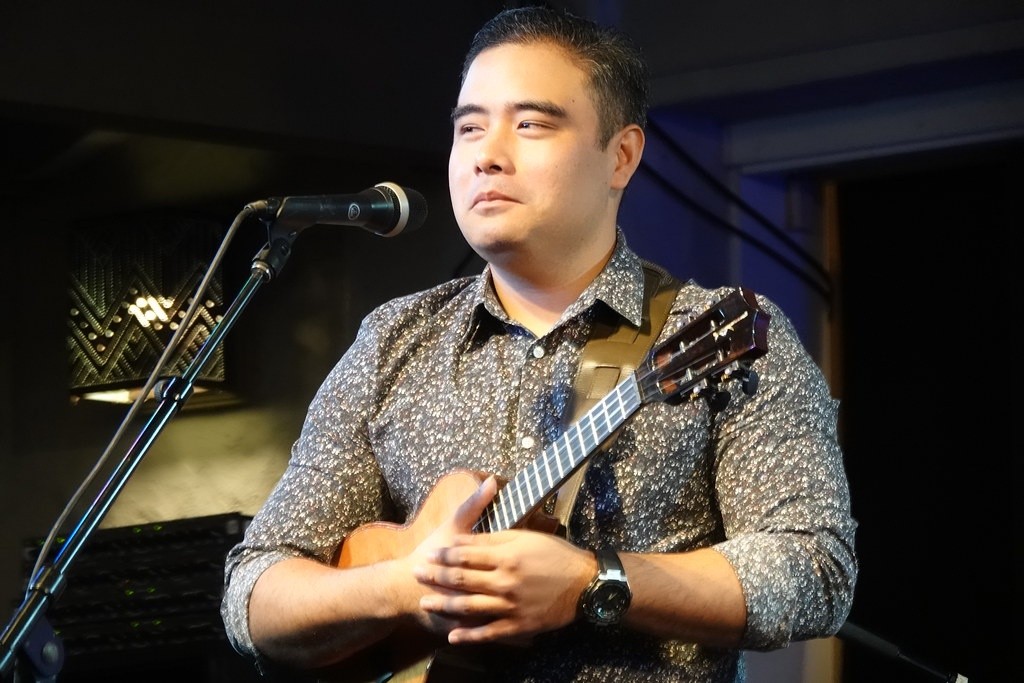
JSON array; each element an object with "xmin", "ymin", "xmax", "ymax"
[{"xmin": 575, "ymin": 552, "xmax": 632, "ymax": 632}]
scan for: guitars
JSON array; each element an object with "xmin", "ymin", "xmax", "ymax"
[{"xmin": 332, "ymin": 286, "xmax": 772, "ymax": 682}]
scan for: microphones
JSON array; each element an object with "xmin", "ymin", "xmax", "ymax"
[{"xmin": 244, "ymin": 180, "xmax": 428, "ymax": 239}]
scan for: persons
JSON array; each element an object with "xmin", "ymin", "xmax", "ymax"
[{"xmin": 218, "ymin": 3, "xmax": 860, "ymax": 683}]
